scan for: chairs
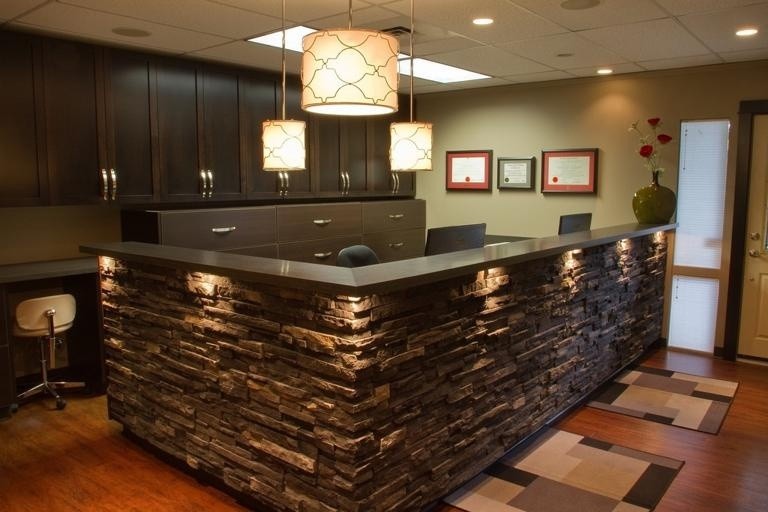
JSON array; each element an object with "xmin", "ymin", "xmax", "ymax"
[{"xmin": 13, "ymin": 293, "xmax": 91, "ymax": 411}]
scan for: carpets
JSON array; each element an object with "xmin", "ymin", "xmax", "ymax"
[
  {"xmin": 444, "ymin": 426, "xmax": 685, "ymax": 512},
  {"xmin": 585, "ymin": 364, "xmax": 740, "ymax": 435}
]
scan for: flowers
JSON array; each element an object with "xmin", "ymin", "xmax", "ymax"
[{"xmin": 628, "ymin": 118, "xmax": 672, "ymax": 171}]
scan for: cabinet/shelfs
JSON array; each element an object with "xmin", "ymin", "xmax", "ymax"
[
  {"xmin": 371, "ymin": 94, "xmax": 416, "ymax": 197},
  {"xmin": 0, "ymin": 256, "xmax": 106, "ymax": 412},
  {"xmin": 0, "ymin": 27, "xmax": 49, "ymax": 207},
  {"xmin": 157, "ymin": 51, "xmax": 246, "ymax": 203},
  {"xmin": 44, "ymin": 35, "xmax": 160, "ymax": 207},
  {"xmin": 314, "ymin": 116, "xmax": 371, "ymax": 197},
  {"xmin": 246, "ymin": 66, "xmax": 315, "ymax": 200}
]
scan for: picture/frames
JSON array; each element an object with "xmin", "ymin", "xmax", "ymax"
[
  {"xmin": 496, "ymin": 156, "xmax": 535, "ymax": 190},
  {"xmin": 540, "ymin": 148, "xmax": 598, "ymax": 193},
  {"xmin": 445, "ymin": 149, "xmax": 493, "ymax": 191}
]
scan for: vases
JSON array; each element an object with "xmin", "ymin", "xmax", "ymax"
[{"xmin": 632, "ymin": 171, "xmax": 676, "ymax": 226}]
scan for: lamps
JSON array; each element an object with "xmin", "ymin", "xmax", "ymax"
[
  {"xmin": 261, "ymin": 1, "xmax": 307, "ymax": 172},
  {"xmin": 389, "ymin": 1, "xmax": 433, "ymax": 171},
  {"xmin": 300, "ymin": 0, "xmax": 399, "ymax": 117}
]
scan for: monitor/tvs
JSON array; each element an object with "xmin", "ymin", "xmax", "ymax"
[
  {"xmin": 558, "ymin": 213, "xmax": 592, "ymax": 235},
  {"xmin": 425, "ymin": 222, "xmax": 486, "ymax": 255}
]
